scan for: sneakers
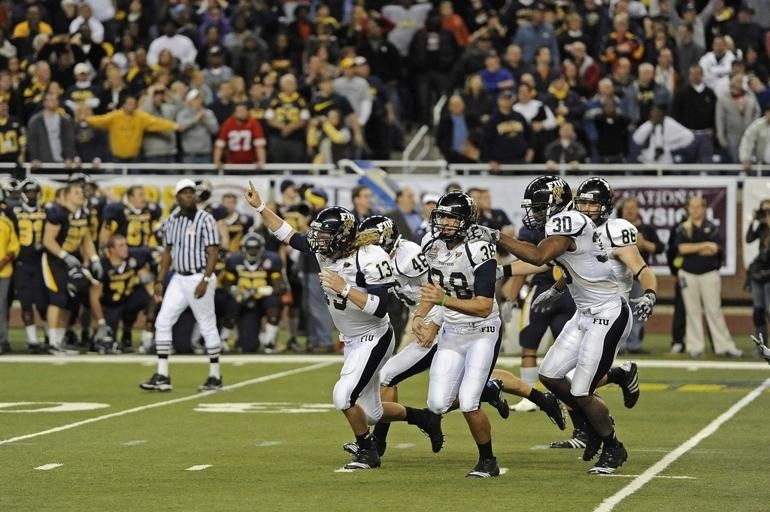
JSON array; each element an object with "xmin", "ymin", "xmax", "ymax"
[
  {"xmin": 487, "ymin": 379, "xmax": 508, "ymax": 418},
  {"xmin": 468, "ymin": 457, "xmax": 498, "ymax": 478},
  {"xmin": 549, "ymin": 430, "xmax": 627, "ymax": 475},
  {"xmin": 196, "ymin": 376, "xmax": 222, "ymax": 389},
  {"xmin": 420, "ymin": 408, "xmax": 445, "ymax": 452},
  {"xmin": 333, "ymin": 442, "xmax": 385, "ymax": 473},
  {"xmin": 139, "ymin": 374, "xmax": 171, "ymax": 391},
  {"xmin": 619, "ymin": 360, "xmax": 639, "ymax": 408},
  {"xmin": 544, "ymin": 392, "xmax": 565, "ymax": 430},
  {"xmin": 510, "ymin": 398, "xmax": 537, "ymax": 411}
]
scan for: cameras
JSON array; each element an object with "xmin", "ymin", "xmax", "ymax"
[
  {"xmin": 654, "ymin": 147, "xmax": 663, "ymax": 160},
  {"xmin": 756, "ymin": 207, "xmax": 770, "ymax": 219}
]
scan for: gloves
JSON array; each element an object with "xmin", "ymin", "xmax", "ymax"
[
  {"xmin": 629, "ymin": 292, "xmax": 656, "ymax": 322},
  {"xmin": 531, "ymin": 287, "xmax": 564, "ymax": 313}
]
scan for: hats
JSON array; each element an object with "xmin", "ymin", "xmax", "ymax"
[{"xmin": 177, "ymin": 180, "xmax": 195, "ymax": 193}]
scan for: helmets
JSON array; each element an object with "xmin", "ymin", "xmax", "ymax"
[
  {"xmin": 307, "ymin": 207, "xmax": 402, "ymax": 257},
  {"xmin": 2, "ymin": 180, "xmax": 41, "ymax": 212},
  {"xmin": 574, "ymin": 178, "xmax": 614, "ymax": 226},
  {"xmin": 521, "ymin": 175, "xmax": 573, "ymax": 231},
  {"xmin": 240, "ymin": 232, "xmax": 266, "ymax": 261},
  {"xmin": 429, "ymin": 192, "xmax": 480, "ymax": 241}
]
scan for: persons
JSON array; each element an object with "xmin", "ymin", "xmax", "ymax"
[
  {"xmin": 0, "ymin": 1, "xmax": 769, "ymax": 357},
  {"xmin": 140, "ymin": 180, "xmax": 224, "ymax": 391},
  {"xmin": 243, "ymin": 177, "xmax": 658, "ymax": 479}
]
